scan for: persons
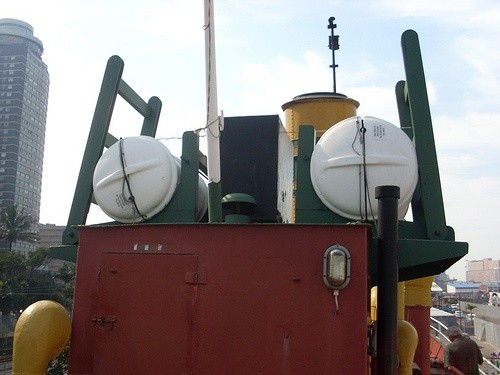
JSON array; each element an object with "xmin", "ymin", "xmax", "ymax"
[{"xmin": 444, "ymin": 326, "xmax": 483, "ymax": 375}]
